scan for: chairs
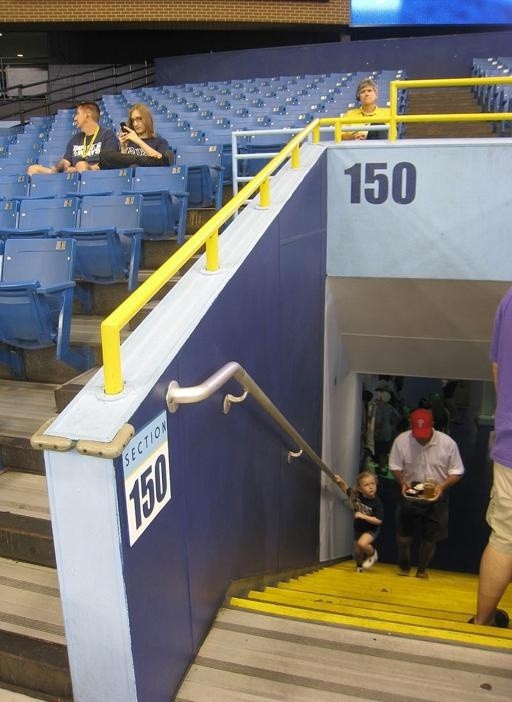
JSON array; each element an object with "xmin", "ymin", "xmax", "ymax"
[{"xmin": 471, "ymin": 56, "xmax": 512, "ymax": 137}]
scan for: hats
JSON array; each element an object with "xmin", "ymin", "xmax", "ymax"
[
  {"xmin": 374, "ymin": 380, "xmax": 388, "ymax": 390},
  {"xmin": 411, "ymin": 409, "xmax": 434, "ymax": 439}
]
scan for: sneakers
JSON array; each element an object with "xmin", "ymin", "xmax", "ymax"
[
  {"xmin": 468, "ymin": 608, "xmax": 509, "ymax": 627},
  {"xmin": 416, "ymin": 569, "xmax": 428, "ymax": 577},
  {"xmin": 399, "ymin": 560, "xmax": 412, "ymax": 576},
  {"xmin": 355, "ymin": 549, "xmax": 378, "ymax": 573}
]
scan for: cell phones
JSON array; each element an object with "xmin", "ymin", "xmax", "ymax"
[{"xmin": 120, "ymin": 122, "xmax": 128, "ymax": 134}]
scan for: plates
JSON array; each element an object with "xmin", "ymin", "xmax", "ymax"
[{"xmin": 401, "ymin": 480, "xmax": 441, "ymax": 501}]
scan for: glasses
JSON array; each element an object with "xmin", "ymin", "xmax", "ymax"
[{"xmin": 130, "ymin": 116, "xmax": 143, "ymax": 124}]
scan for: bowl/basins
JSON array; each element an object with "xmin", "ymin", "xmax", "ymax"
[
  {"xmin": 413, "ymin": 483, "xmax": 424, "ymax": 495},
  {"xmin": 405, "ymin": 488, "xmax": 418, "ymax": 497}
]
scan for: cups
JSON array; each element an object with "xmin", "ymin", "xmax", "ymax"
[{"xmin": 423, "ymin": 483, "xmax": 436, "ymax": 500}]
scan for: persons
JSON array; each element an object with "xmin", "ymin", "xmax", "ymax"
[
  {"xmin": 117, "ymin": 103, "xmax": 173, "ymax": 167},
  {"xmin": 339, "ymin": 78, "xmax": 391, "ymax": 140},
  {"xmin": 466, "ymin": 288, "xmax": 512, "ymax": 628},
  {"xmin": 28, "ymin": 101, "xmax": 118, "ymax": 178},
  {"xmin": 352, "ymin": 375, "xmax": 464, "ymax": 580}
]
